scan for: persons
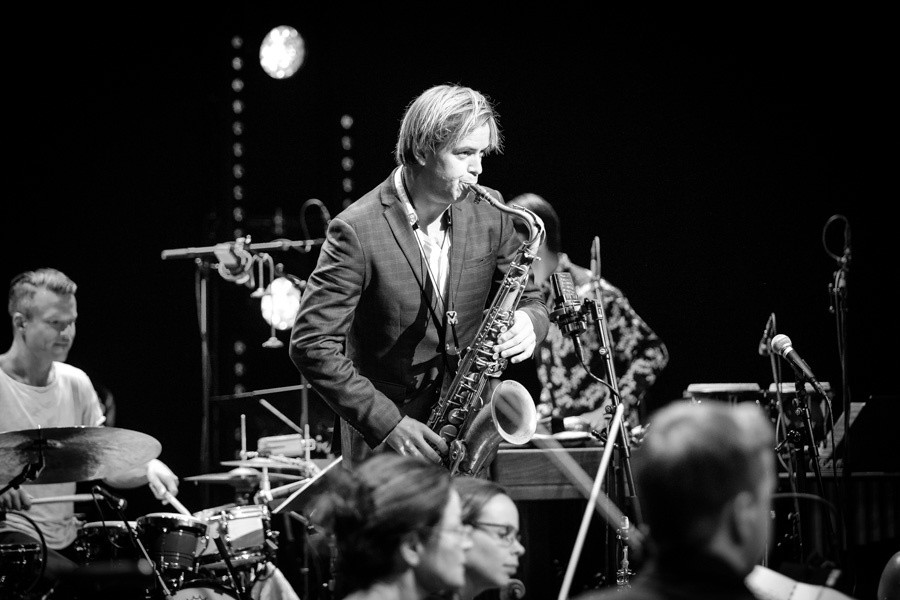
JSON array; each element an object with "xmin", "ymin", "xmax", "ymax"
[
  {"xmin": 0, "ymin": 268, "xmax": 180, "ymax": 581},
  {"xmin": 304, "ymin": 453, "xmax": 527, "ymax": 600},
  {"xmin": 506, "ymin": 193, "xmax": 669, "ymax": 447},
  {"xmin": 288, "ymin": 84, "xmax": 550, "ymax": 468},
  {"xmin": 573, "ymin": 397, "xmax": 778, "ymax": 600}
]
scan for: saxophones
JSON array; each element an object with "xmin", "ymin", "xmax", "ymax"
[{"xmin": 417, "ymin": 182, "xmax": 550, "ymax": 480}]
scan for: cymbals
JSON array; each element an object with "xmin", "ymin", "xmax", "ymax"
[
  {"xmin": 183, "ymin": 467, "xmax": 305, "ymax": 483},
  {"xmin": 0, "ymin": 425, "xmax": 163, "ymax": 486},
  {"xmin": 219, "ymin": 457, "xmax": 301, "ymax": 470}
]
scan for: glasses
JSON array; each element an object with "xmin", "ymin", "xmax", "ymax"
[{"xmin": 471, "ymin": 520, "xmax": 521, "ymax": 544}]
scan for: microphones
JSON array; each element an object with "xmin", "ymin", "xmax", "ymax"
[
  {"xmin": 550, "ymin": 272, "xmax": 586, "ymax": 360},
  {"xmin": 590, "ymin": 241, "xmax": 599, "ymax": 282},
  {"xmin": 759, "ymin": 316, "xmax": 775, "ymax": 356},
  {"xmin": 843, "ymin": 224, "xmax": 851, "ymax": 262},
  {"xmin": 772, "ymin": 333, "xmax": 824, "ymax": 394},
  {"xmin": 97, "ymin": 486, "xmax": 127, "ymax": 510}
]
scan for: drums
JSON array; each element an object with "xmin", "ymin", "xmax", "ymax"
[
  {"xmin": 191, "ymin": 504, "xmax": 270, "ymax": 570},
  {"xmin": 80, "ymin": 520, "xmax": 139, "ymax": 565},
  {"xmin": 138, "ymin": 513, "xmax": 208, "ymax": 567},
  {"xmin": 687, "ymin": 379, "xmax": 834, "ymax": 468}
]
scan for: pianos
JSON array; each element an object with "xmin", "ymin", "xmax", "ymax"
[{"xmin": 489, "ymin": 441, "xmax": 846, "ymax": 594}]
